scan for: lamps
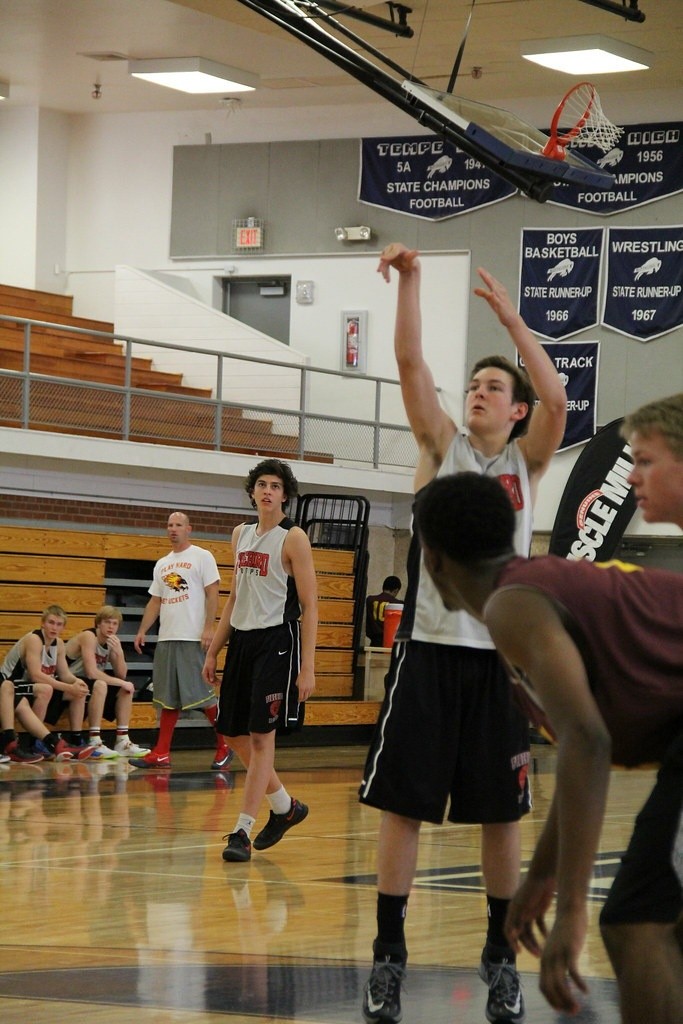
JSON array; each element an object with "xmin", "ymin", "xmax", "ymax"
[{"xmin": 335, "ymin": 227, "xmax": 372, "ymax": 242}]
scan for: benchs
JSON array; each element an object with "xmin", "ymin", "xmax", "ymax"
[{"xmin": 0, "ymin": 281, "xmax": 381, "ymax": 734}]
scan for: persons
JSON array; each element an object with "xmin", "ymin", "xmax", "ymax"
[
  {"xmin": 55, "ymin": 605, "xmax": 151, "ymax": 758},
  {"xmin": 357, "ymin": 243, "xmax": 567, "ymax": 1024},
  {"xmin": 411, "ymin": 472, "xmax": 683, "ymax": 1024},
  {"xmin": 0, "ymin": 605, "xmax": 103, "ymax": 760},
  {"xmin": 619, "ymin": 393, "xmax": 683, "ymax": 883},
  {"xmin": 366, "ymin": 576, "xmax": 405, "ymax": 654},
  {"xmin": 0, "ymin": 672, "xmax": 99, "ymax": 765},
  {"xmin": 0, "ymin": 754, "xmax": 11, "ymax": 762},
  {"xmin": 129, "ymin": 512, "xmax": 234, "ymax": 770},
  {"xmin": 201, "ymin": 459, "xmax": 319, "ymax": 862}
]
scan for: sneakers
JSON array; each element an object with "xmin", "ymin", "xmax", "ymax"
[
  {"xmin": 211, "ymin": 748, "xmax": 233, "ymax": 770},
  {"xmin": 0, "ymin": 735, "xmax": 152, "ymax": 764},
  {"xmin": 0, "ymin": 756, "xmax": 139, "ymax": 777},
  {"xmin": 215, "ymin": 769, "xmax": 233, "ymax": 793},
  {"xmin": 223, "ymin": 828, "xmax": 252, "ymax": 862},
  {"xmin": 254, "ymin": 797, "xmax": 309, "ymax": 851},
  {"xmin": 223, "ymin": 860, "xmax": 251, "ymax": 889},
  {"xmin": 361, "ymin": 940, "xmax": 408, "ymax": 1024},
  {"xmin": 252, "ymin": 856, "xmax": 305, "ymax": 908},
  {"xmin": 128, "ymin": 767, "xmax": 171, "ymax": 783},
  {"xmin": 480, "ymin": 947, "xmax": 527, "ymax": 1024},
  {"xmin": 128, "ymin": 753, "xmax": 172, "ymax": 768}
]
